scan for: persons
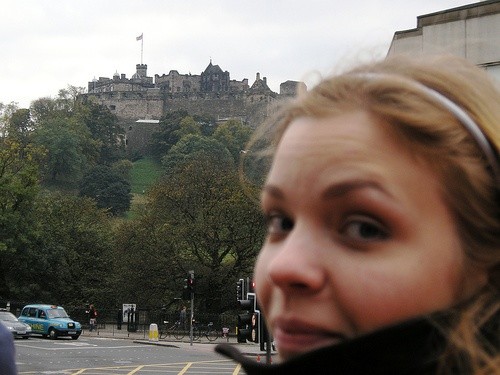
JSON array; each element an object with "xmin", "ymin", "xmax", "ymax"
[
  {"xmin": 85, "ymin": 304, "xmax": 97, "ymax": 332},
  {"xmin": 40, "ymin": 312, "xmax": 45, "ymax": 318},
  {"xmin": 180, "ymin": 306, "xmax": 187, "ymax": 335},
  {"xmin": 214, "ymin": 44, "xmax": 500, "ymax": 375}
]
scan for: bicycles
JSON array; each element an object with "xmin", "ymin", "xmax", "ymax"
[
  {"xmin": 158, "ymin": 318, "xmax": 188, "ymax": 341},
  {"xmin": 186, "ymin": 321, "xmax": 219, "ymax": 341}
]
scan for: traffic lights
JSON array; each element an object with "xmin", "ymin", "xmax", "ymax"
[
  {"xmin": 237, "ymin": 292, "xmax": 260, "ymax": 344},
  {"xmin": 236, "ymin": 279, "xmax": 244, "ymax": 302},
  {"xmin": 188, "ymin": 277, "xmax": 194, "ymax": 287}
]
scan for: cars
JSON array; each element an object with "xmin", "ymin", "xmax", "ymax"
[{"xmin": 0, "ymin": 308, "xmax": 33, "ymax": 339}]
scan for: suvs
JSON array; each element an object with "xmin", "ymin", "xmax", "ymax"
[{"xmin": 18, "ymin": 303, "xmax": 83, "ymax": 340}]
